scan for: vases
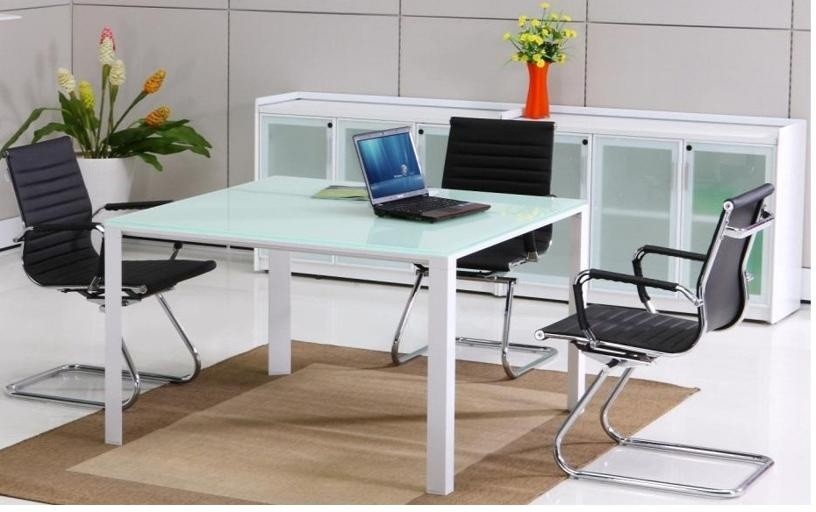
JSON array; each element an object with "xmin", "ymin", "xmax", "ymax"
[
  {"xmin": 74, "ymin": 151, "xmax": 139, "ymax": 221},
  {"xmin": 522, "ymin": 59, "xmax": 553, "ymax": 120}
]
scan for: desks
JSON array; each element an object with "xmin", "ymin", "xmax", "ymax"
[{"xmin": 99, "ymin": 171, "xmax": 592, "ymax": 497}]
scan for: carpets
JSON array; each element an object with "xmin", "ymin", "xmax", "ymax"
[{"xmin": 0, "ymin": 333, "xmax": 702, "ymax": 505}]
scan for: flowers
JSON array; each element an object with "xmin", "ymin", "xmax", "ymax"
[
  {"xmin": 0, "ymin": 19, "xmax": 213, "ymax": 171},
  {"xmin": 495, "ymin": 2, "xmax": 579, "ymax": 70}
]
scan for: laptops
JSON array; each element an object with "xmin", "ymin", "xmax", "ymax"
[{"xmin": 353, "ymin": 126, "xmax": 491, "ymax": 223}]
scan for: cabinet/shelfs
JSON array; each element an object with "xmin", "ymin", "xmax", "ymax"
[
  {"xmin": 592, "ymin": 107, "xmax": 807, "ymax": 326},
  {"xmin": 414, "ymin": 102, "xmax": 591, "ymax": 304},
  {"xmin": 250, "ymin": 92, "xmax": 414, "ymax": 288}
]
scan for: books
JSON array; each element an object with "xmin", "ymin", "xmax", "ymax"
[{"xmin": 311, "ymin": 184, "xmax": 370, "ymax": 201}]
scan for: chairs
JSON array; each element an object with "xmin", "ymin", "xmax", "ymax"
[
  {"xmin": 1, "ymin": 135, "xmax": 219, "ymax": 410},
  {"xmin": 388, "ymin": 116, "xmax": 559, "ymax": 381},
  {"xmin": 532, "ymin": 182, "xmax": 778, "ymax": 500}
]
